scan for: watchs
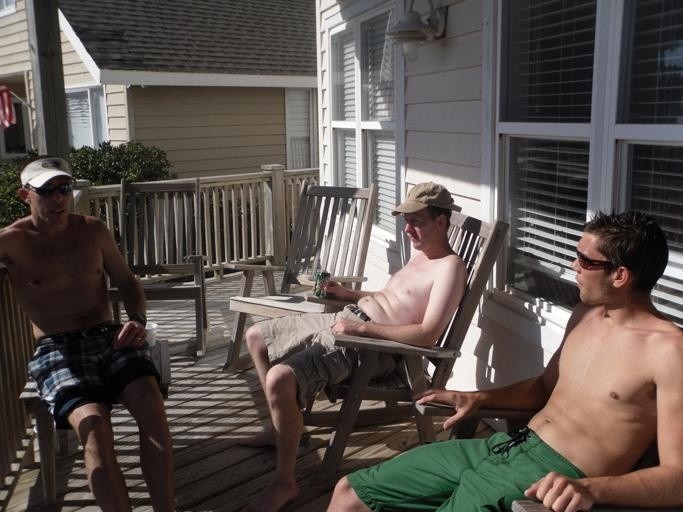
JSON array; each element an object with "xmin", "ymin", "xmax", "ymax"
[{"xmin": 129, "ymin": 311, "xmax": 148, "ymax": 324}]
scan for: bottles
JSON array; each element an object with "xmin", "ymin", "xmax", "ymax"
[{"xmin": 314, "ymin": 269, "xmax": 330, "ymax": 298}]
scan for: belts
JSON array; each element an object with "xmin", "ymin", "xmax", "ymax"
[
  {"xmin": 346, "ymin": 305, "xmax": 405, "ymax": 363},
  {"xmin": 29, "ymin": 320, "xmax": 120, "ymax": 348}
]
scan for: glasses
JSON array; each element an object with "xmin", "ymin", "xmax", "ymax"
[
  {"xmin": 25, "ymin": 183, "xmax": 77, "ymax": 198},
  {"xmin": 576, "ymin": 248, "xmax": 621, "ymax": 271}
]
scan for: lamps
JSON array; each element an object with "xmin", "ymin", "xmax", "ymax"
[{"xmin": 386, "ymin": 0, "xmax": 448, "ymax": 61}]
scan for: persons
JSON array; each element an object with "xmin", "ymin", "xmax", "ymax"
[
  {"xmin": 238, "ymin": 182, "xmax": 467, "ymax": 511},
  {"xmin": 325, "ymin": 211, "xmax": 681, "ymax": 511},
  {"xmin": 2, "ymin": 155, "xmax": 177, "ymax": 511}
]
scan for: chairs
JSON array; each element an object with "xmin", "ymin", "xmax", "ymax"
[
  {"xmin": 303, "ymin": 220, "xmax": 510, "ymax": 479},
  {"xmin": 107, "ymin": 177, "xmax": 207, "ymax": 357},
  {"xmin": 224, "ymin": 180, "xmax": 376, "ymax": 372},
  {"xmin": 416, "ymin": 403, "xmax": 683, "ymax": 512}
]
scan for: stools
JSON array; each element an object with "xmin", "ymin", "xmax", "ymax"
[{"xmin": 19, "ymin": 340, "xmax": 171, "ymax": 505}]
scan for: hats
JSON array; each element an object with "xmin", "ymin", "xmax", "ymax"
[
  {"xmin": 391, "ymin": 181, "xmax": 462, "ymax": 217},
  {"xmin": 19, "ymin": 155, "xmax": 79, "ymax": 190}
]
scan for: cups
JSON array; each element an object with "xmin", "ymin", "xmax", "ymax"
[{"xmin": 144, "ymin": 322, "xmax": 158, "ymax": 347}]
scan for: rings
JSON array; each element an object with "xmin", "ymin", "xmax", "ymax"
[{"xmin": 134, "ymin": 336, "xmax": 142, "ymax": 340}]
